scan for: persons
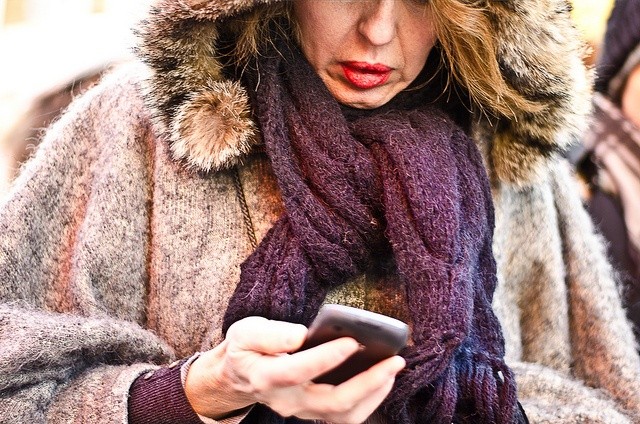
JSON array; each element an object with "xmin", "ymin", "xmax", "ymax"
[
  {"xmin": 571, "ymin": 0, "xmax": 639, "ymax": 356},
  {"xmin": 0, "ymin": 0, "xmax": 640, "ymax": 424}
]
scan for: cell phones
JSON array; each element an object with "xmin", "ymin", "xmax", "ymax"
[{"xmin": 302, "ymin": 303, "xmax": 410, "ymax": 385}]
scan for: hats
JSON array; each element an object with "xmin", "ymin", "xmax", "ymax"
[{"xmin": 592, "ymin": 0, "xmax": 640, "ymax": 101}]
